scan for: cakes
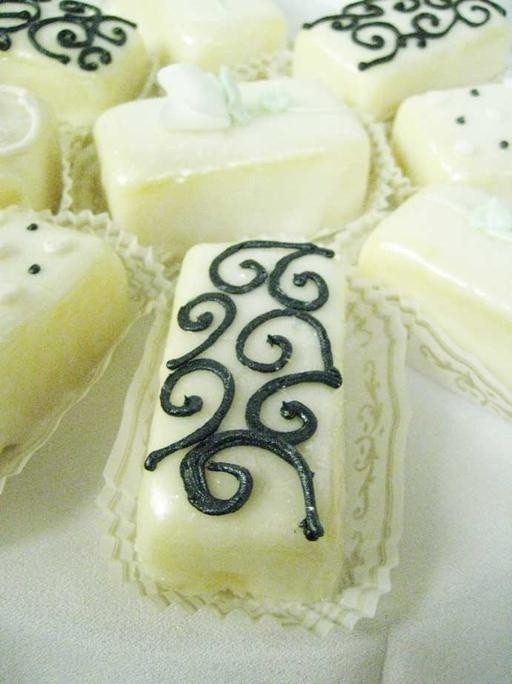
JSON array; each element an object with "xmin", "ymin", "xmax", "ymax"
[{"xmin": 1, "ymin": 1, "xmax": 512, "ymax": 606}]
